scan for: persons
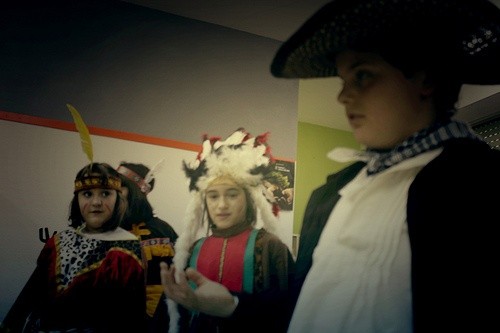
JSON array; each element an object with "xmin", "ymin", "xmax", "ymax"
[
  {"xmin": 176, "ymin": 171, "xmax": 295, "ymax": 333},
  {"xmin": 0, "ymin": 163, "xmax": 147, "ymax": 333},
  {"xmin": 115, "ymin": 163, "xmax": 179, "ymax": 333},
  {"xmin": 160, "ymin": 0, "xmax": 500, "ymax": 333}
]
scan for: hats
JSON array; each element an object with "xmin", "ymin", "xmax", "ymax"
[{"xmin": 270, "ymin": 0, "xmax": 500, "ymax": 86}]
